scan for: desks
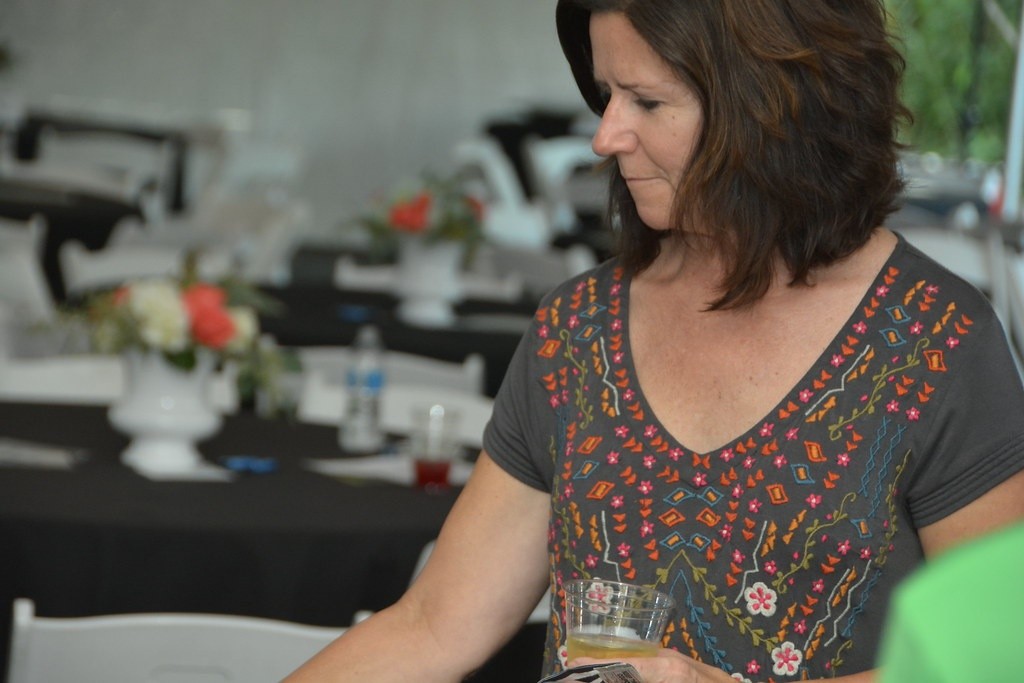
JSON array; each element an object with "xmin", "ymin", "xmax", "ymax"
[
  {"xmin": 0, "ymin": 394, "xmax": 544, "ymax": 683},
  {"xmin": 290, "ymin": 253, "xmax": 548, "ymax": 392}
]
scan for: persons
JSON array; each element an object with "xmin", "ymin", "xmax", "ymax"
[{"xmin": 279, "ymin": 2, "xmax": 1023, "ymax": 682}]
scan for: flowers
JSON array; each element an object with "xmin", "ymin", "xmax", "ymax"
[
  {"xmin": 63, "ymin": 274, "xmax": 304, "ymax": 422},
  {"xmin": 344, "ymin": 158, "xmax": 497, "ymax": 276}
]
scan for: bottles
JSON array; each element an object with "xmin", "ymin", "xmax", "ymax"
[{"xmin": 339, "ymin": 327, "xmax": 386, "ymax": 451}]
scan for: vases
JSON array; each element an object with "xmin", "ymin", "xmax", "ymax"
[
  {"xmin": 115, "ymin": 350, "xmax": 239, "ymax": 490},
  {"xmin": 391, "ymin": 235, "xmax": 469, "ymax": 325}
]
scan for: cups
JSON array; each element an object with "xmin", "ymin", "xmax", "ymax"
[
  {"xmin": 409, "ymin": 407, "xmax": 457, "ymax": 489},
  {"xmin": 561, "ymin": 579, "xmax": 677, "ymax": 667}
]
